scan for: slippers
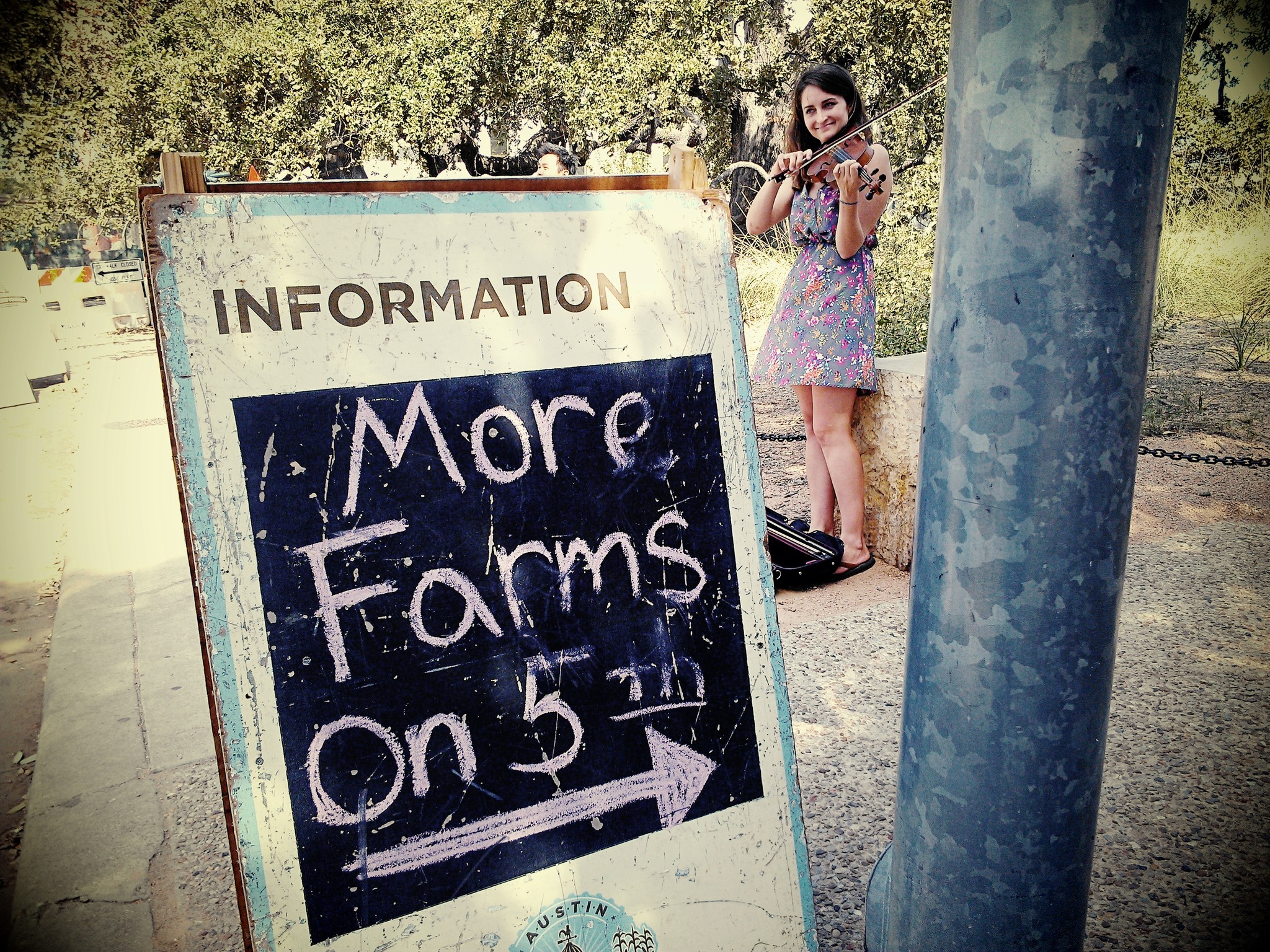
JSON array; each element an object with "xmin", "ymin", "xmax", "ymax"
[{"xmin": 827, "ymin": 556, "xmax": 876, "ymax": 582}]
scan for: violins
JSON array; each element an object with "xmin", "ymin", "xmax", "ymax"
[{"xmin": 806, "ymin": 126, "xmax": 887, "ymax": 200}]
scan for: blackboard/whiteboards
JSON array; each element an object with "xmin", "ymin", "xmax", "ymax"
[{"xmin": 142, "ymin": 158, "xmax": 819, "ymax": 952}]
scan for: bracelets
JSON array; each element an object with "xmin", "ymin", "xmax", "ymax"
[{"xmin": 839, "ymin": 197, "xmax": 859, "ymax": 205}]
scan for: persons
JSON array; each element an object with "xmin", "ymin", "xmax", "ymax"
[
  {"xmin": 745, "ymin": 64, "xmax": 894, "ymax": 587},
  {"xmin": 535, "ymin": 141, "xmax": 578, "ymax": 176}
]
